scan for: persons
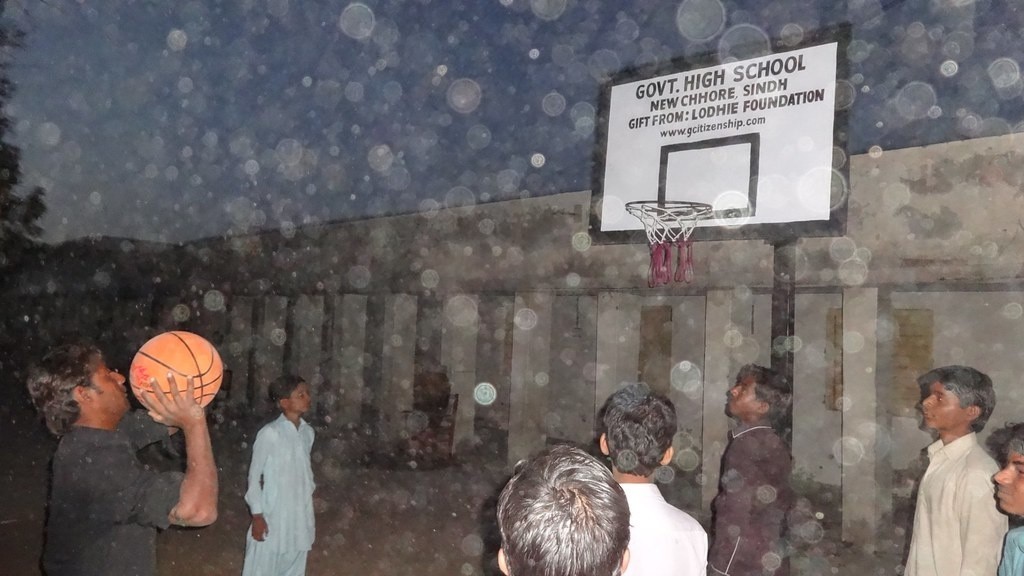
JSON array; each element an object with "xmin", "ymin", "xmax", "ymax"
[
  {"xmin": 242, "ymin": 372, "xmax": 317, "ymax": 576},
  {"xmin": 707, "ymin": 364, "xmax": 794, "ymax": 576},
  {"xmin": 497, "ymin": 444, "xmax": 632, "ymax": 576},
  {"xmin": 24, "ymin": 339, "xmax": 219, "ymax": 576},
  {"xmin": 901, "ymin": 367, "xmax": 1009, "ymax": 576},
  {"xmin": 600, "ymin": 382, "xmax": 708, "ymax": 576},
  {"xmin": 988, "ymin": 420, "xmax": 1024, "ymax": 576}
]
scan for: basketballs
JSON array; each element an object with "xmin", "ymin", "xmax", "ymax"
[{"xmin": 129, "ymin": 329, "xmax": 223, "ymax": 412}]
